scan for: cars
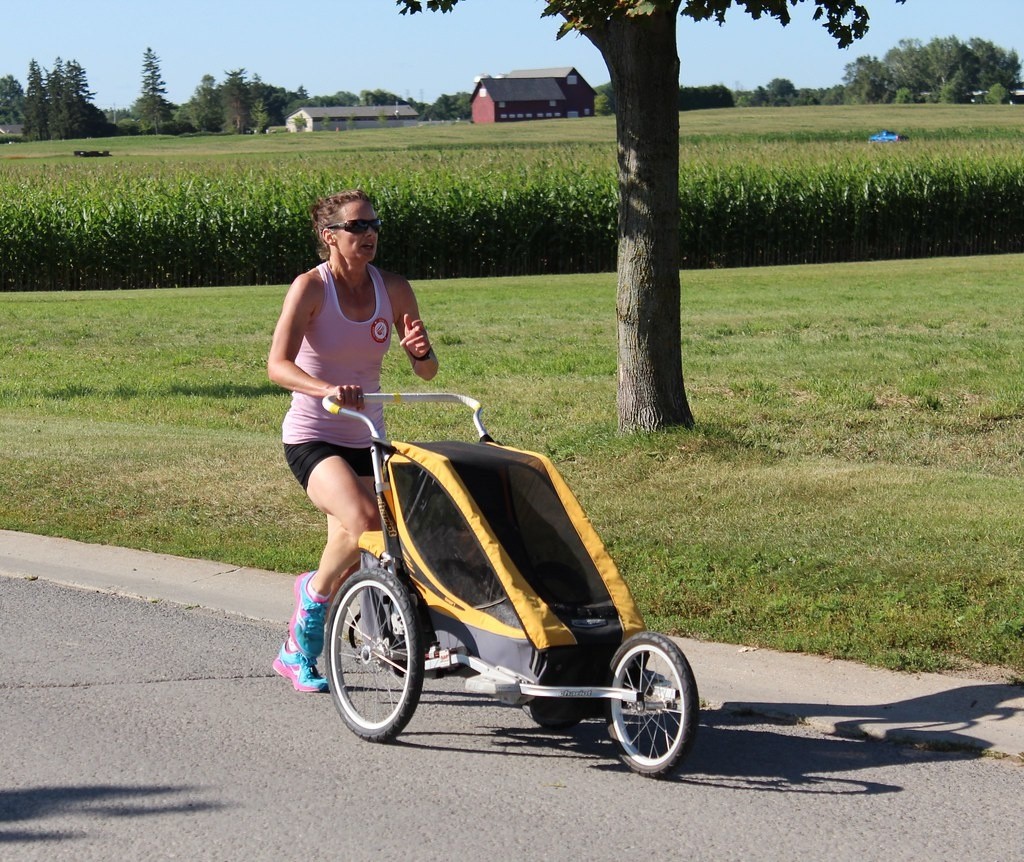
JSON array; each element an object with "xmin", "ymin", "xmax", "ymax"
[{"xmin": 868, "ymin": 131, "xmax": 910, "ymax": 143}]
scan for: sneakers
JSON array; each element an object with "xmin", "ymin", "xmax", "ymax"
[
  {"xmin": 290, "ymin": 570, "xmax": 334, "ymax": 661},
  {"xmin": 271, "ymin": 640, "xmax": 329, "ymax": 692}
]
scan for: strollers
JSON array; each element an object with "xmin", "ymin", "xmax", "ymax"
[{"xmin": 326, "ymin": 391, "xmax": 699, "ymax": 778}]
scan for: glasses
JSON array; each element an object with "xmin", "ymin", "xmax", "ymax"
[{"xmin": 320, "ymin": 219, "xmax": 382, "ymax": 234}]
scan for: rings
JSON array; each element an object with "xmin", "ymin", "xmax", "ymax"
[{"xmin": 357, "ymin": 395, "xmax": 364, "ymax": 398}]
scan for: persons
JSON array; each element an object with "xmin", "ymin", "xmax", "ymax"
[{"xmin": 267, "ymin": 190, "xmax": 440, "ymax": 692}]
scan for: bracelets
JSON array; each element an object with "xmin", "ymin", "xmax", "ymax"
[{"xmin": 409, "ymin": 349, "xmax": 433, "ymax": 361}]
